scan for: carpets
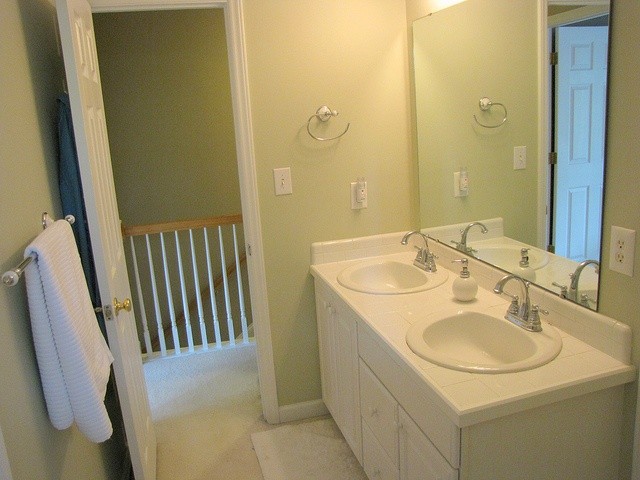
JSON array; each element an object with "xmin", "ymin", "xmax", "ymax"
[{"xmin": 251, "ymin": 419, "xmax": 367, "ymax": 479}]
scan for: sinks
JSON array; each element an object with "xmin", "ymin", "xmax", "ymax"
[
  {"xmin": 339, "ymin": 262, "xmax": 447, "ymax": 295},
  {"xmin": 406, "ymin": 310, "xmax": 564, "ymax": 374},
  {"xmin": 477, "ymin": 246, "xmax": 545, "ymax": 270}
]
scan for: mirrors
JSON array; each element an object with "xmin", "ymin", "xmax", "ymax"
[{"xmin": 413, "ymin": 1, "xmax": 612, "ymax": 312}]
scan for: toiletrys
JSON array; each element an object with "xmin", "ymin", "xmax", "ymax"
[
  {"xmin": 516, "ymin": 246, "xmax": 536, "ymax": 284},
  {"xmin": 452, "ymin": 259, "xmax": 478, "ymax": 301}
]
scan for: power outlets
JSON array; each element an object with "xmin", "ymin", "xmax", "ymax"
[
  {"xmin": 452, "ymin": 167, "xmax": 470, "ymax": 198},
  {"xmin": 607, "ymin": 223, "xmax": 636, "ymax": 275},
  {"xmin": 350, "ymin": 181, "xmax": 368, "ymax": 209}
]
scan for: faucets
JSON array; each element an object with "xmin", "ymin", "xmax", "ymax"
[
  {"xmin": 452, "ymin": 221, "xmax": 488, "ymax": 255},
  {"xmin": 401, "ymin": 229, "xmax": 438, "ymax": 274},
  {"xmin": 494, "ymin": 274, "xmax": 549, "ymax": 333},
  {"xmin": 553, "ymin": 259, "xmax": 599, "ymax": 308}
]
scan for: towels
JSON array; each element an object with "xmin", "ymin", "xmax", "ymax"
[{"xmin": 23, "ymin": 220, "xmax": 114, "ymax": 445}]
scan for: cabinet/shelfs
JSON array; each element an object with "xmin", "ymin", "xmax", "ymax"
[
  {"xmin": 357, "ymin": 319, "xmax": 462, "ymax": 479},
  {"xmin": 312, "ymin": 277, "xmax": 362, "ymax": 470}
]
